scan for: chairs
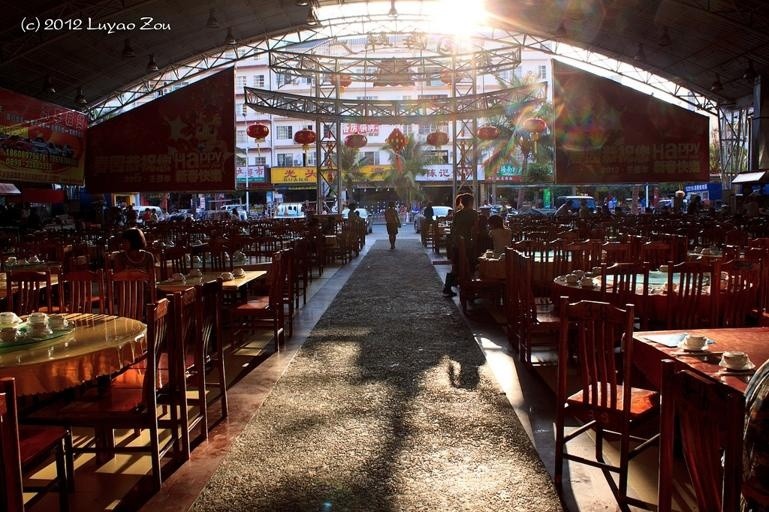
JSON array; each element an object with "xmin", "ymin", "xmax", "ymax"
[
  {"xmin": 0, "ymin": 203, "xmax": 366, "ymax": 511},
  {"xmin": 414, "ymin": 195, "xmax": 769, "ymax": 512}
]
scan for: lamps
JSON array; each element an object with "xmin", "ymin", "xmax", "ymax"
[
  {"xmin": 41, "ymin": 0, "xmax": 401, "ymax": 105},
  {"xmin": 553, "ymin": 17, "xmax": 759, "ymax": 93}
]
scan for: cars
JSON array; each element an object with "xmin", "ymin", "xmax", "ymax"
[
  {"xmin": 625, "ymin": 198, "xmax": 687, "ymax": 214},
  {"xmin": 122, "ymin": 201, "xmax": 305, "ymax": 223},
  {"xmin": 479, "ymin": 202, "xmax": 555, "ymax": 218},
  {"xmin": 412, "ymin": 205, "xmax": 453, "ymax": 233},
  {"xmin": 0, "ymin": 131, "xmax": 75, "ymax": 162},
  {"xmin": 340, "ymin": 209, "xmax": 374, "ymax": 235}
]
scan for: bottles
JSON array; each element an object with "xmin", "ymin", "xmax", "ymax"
[{"xmin": 566, "ymin": 270, "xmax": 593, "ymax": 287}]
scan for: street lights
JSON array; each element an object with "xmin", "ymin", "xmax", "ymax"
[{"xmin": 241, "ymin": 111, "xmax": 248, "ymax": 213}]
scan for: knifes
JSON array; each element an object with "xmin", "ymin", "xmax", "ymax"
[
  {"xmin": 710, "ymin": 371, "xmax": 755, "ymax": 376},
  {"xmin": 671, "ymin": 352, "xmax": 724, "ymax": 355}
]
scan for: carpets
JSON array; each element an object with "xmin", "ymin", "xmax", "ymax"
[{"xmin": 183, "ymin": 238, "xmax": 565, "ymax": 511}]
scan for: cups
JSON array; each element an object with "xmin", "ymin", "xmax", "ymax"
[
  {"xmin": 723, "ymin": 351, "xmax": 749, "ymax": 368},
  {"xmin": 0, "ymin": 311, "xmax": 65, "ymax": 343},
  {"xmin": 664, "ymin": 283, "xmax": 677, "ymax": 290},
  {"xmin": 685, "ymin": 333, "xmax": 708, "ymax": 347},
  {"xmin": 660, "ymin": 265, "xmax": 668, "ymax": 272},
  {"xmin": 721, "ymin": 271, "xmax": 728, "ymax": 290},
  {"xmin": 170, "ymin": 251, "xmax": 247, "ymax": 282},
  {"xmin": 486, "ymin": 251, "xmax": 505, "ymax": 260}
]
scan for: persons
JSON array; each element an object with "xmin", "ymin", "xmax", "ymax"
[
  {"xmin": 555, "ymin": 195, "xmax": 769, "ymax": 238},
  {"xmin": 443, "ymin": 193, "xmax": 513, "ymax": 296},
  {"xmin": 285, "ymin": 201, "xmax": 435, "ymax": 256},
  {"xmin": 0, "ymin": 201, "xmax": 44, "ymax": 232},
  {"xmin": 721, "ymin": 358, "xmax": 768, "ymax": 511},
  {"xmin": 114, "ymin": 229, "xmax": 156, "ymax": 304},
  {"xmin": 103, "ymin": 203, "xmax": 272, "ymax": 225}
]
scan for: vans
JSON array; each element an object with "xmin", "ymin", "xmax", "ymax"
[{"xmin": 555, "ymin": 195, "xmax": 598, "ymax": 214}]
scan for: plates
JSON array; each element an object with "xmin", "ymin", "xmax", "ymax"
[
  {"xmin": 661, "ymin": 286, "xmax": 684, "ymax": 293},
  {"xmin": 677, "ymin": 343, "xmax": 709, "ymax": 351},
  {"xmin": 719, "ymin": 361, "xmax": 757, "ymax": 370}
]
